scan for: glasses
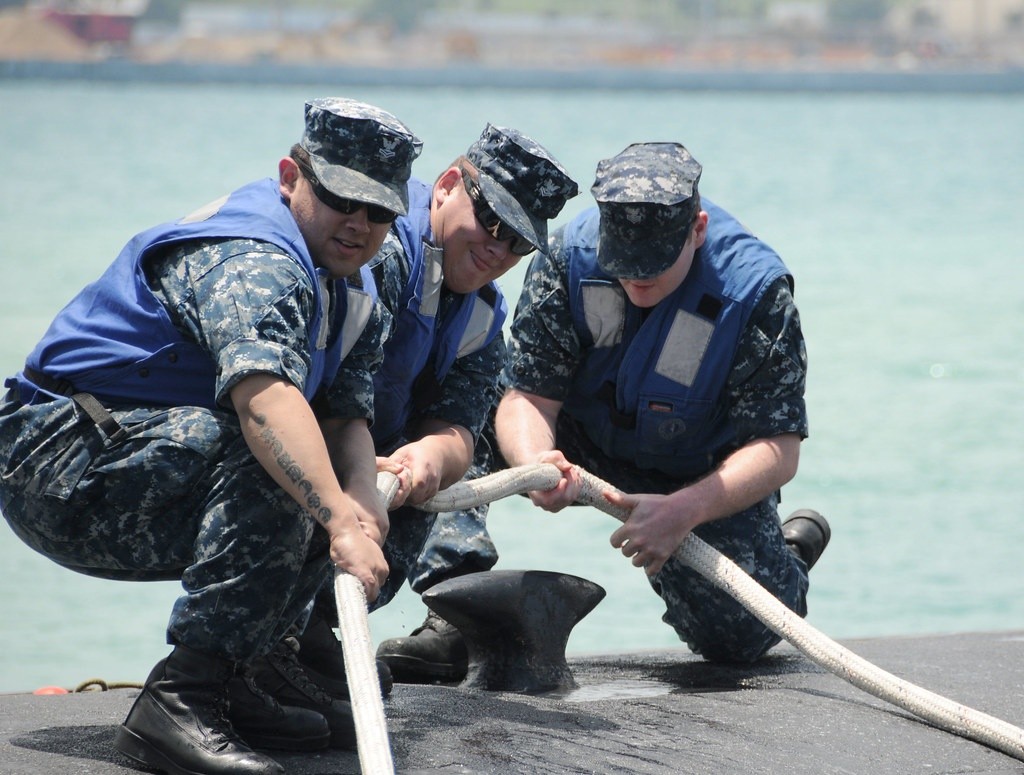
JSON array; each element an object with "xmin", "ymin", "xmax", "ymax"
[
  {"xmin": 290, "ymin": 154, "xmax": 399, "ymax": 223},
  {"xmin": 459, "ymin": 164, "xmax": 539, "ymax": 256}
]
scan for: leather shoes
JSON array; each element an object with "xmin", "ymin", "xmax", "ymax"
[
  {"xmin": 226, "ymin": 658, "xmax": 334, "ymax": 752},
  {"xmin": 298, "ymin": 618, "xmax": 394, "ymax": 700},
  {"xmin": 375, "ymin": 604, "xmax": 469, "ymax": 683},
  {"xmin": 781, "ymin": 507, "xmax": 831, "ymax": 571},
  {"xmin": 112, "ymin": 627, "xmax": 285, "ymax": 773},
  {"xmin": 254, "ymin": 645, "xmax": 360, "ymax": 747}
]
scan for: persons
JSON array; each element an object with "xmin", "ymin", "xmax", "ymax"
[
  {"xmin": 0, "ymin": 94, "xmax": 422, "ymax": 775},
  {"xmin": 377, "ymin": 139, "xmax": 833, "ymax": 691},
  {"xmin": 262, "ymin": 118, "xmax": 581, "ymax": 750}
]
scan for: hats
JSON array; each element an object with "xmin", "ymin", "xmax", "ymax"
[
  {"xmin": 466, "ymin": 122, "xmax": 582, "ymax": 256},
  {"xmin": 299, "ymin": 97, "xmax": 424, "ymax": 216},
  {"xmin": 590, "ymin": 140, "xmax": 702, "ymax": 279}
]
